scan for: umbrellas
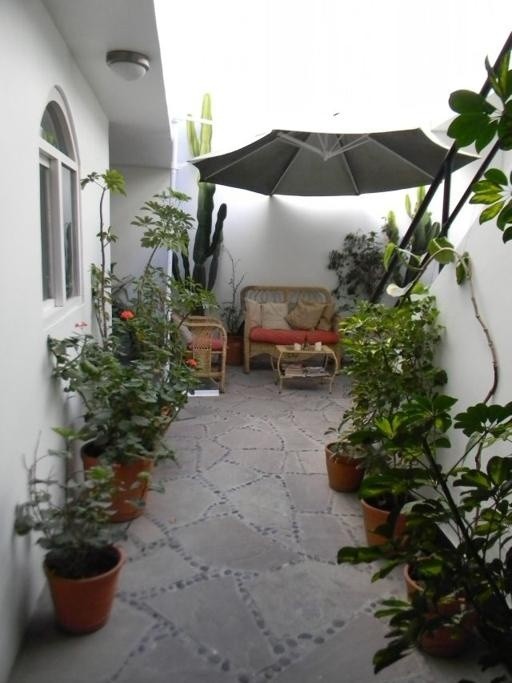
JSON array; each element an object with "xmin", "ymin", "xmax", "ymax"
[{"xmin": 161, "ymin": 102, "xmax": 482, "ymax": 386}]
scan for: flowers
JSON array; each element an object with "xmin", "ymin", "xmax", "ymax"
[{"xmin": 49, "ymin": 309, "xmax": 201, "ymax": 465}]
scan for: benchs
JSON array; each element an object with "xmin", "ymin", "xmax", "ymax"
[{"xmin": 241, "ymin": 287, "xmax": 343, "ymax": 374}]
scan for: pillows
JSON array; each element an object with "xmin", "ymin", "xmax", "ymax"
[{"xmin": 246, "ymin": 297, "xmax": 336, "ymax": 330}]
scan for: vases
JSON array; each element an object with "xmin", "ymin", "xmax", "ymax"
[{"xmin": 80, "ymin": 439, "xmax": 151, "ymax": 522}]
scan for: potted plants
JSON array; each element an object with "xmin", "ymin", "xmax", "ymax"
[
  {"xmin": 10, "ymin": 424, "xmax": 163, "ymax": 637},
  {"xmin": 324, "ymin": 284, "xmax": 512, "ymax": 673}
]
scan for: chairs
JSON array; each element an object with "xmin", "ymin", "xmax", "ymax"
[{"xmin": 172, "ymin": 310, "xmax": 227, "ymax": 395}]
